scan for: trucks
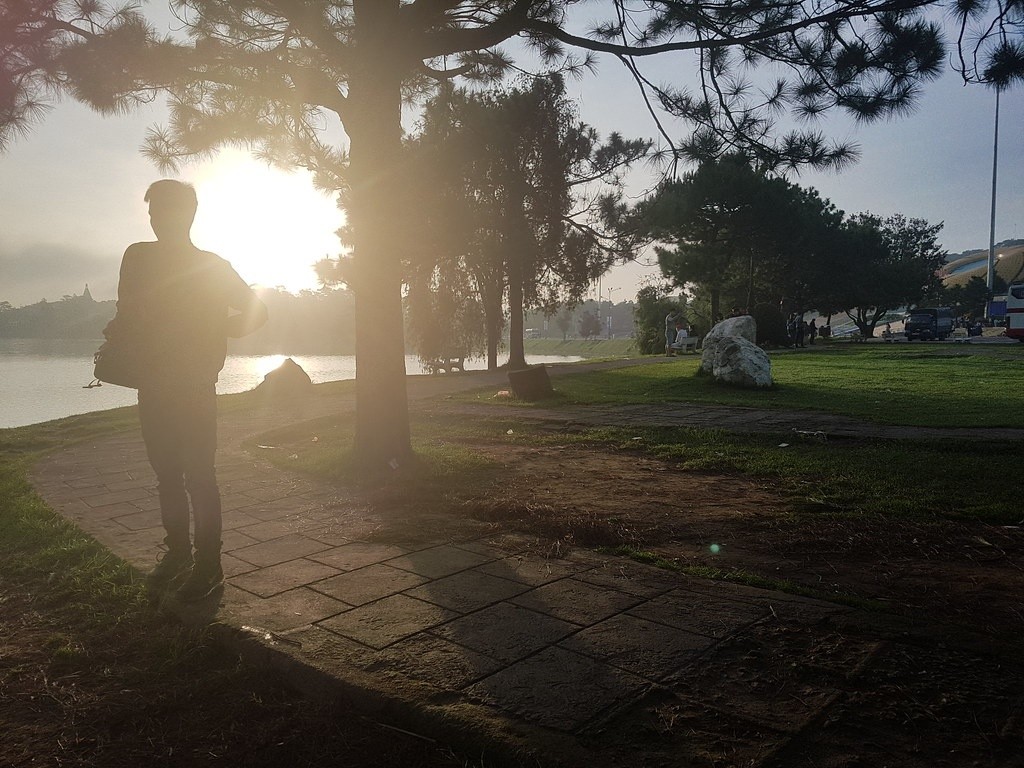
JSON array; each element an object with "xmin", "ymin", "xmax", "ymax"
[
  {"xmin": 902, "ymin": 306, "xmax": 954, "ymax": 342},
  {"xmin": 985, "ymin": 301, "xmax": 1006, "ymax": 326}
]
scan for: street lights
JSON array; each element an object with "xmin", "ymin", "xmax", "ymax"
[{"xmin": 601, "ymin": 288, "xmax": 621, "ymax": 339}]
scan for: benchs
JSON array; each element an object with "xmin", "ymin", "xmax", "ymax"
[
  {"xmin": 952, "ymin": 333, "xmax": 972, "ymax": 344},
  {"xmin": 431, "ymin": 349, "xmax": 468, "ymax": 374},
  {"xmin": 665, "ymin": 336, "xmax": 698, "ymax": 355}
]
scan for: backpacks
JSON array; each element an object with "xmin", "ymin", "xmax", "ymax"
[{"xmin": 92, "ymin": 300, "xmax": 141, "ymax": 389}]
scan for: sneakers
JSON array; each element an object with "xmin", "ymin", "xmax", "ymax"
[
  {"xmin": 175, "ymin": 560, "xmax": 227, "ymax": 603},
  {"xmin": 147, "ymin": 543, "xmax": 195, "ymax": 585}
]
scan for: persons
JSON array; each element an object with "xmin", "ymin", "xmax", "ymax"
[
  {"xmin": 101, "ymin": 179, "xmax": 270, "ymax": 601},
  {"xmin": 885, "ymin": 322, "xmax": 894, "ymax": 339},
  {"xmin": 687, "ymin": 325, "xmax": 696, "ymax": 347},
  {"xmin": 664, "ymin": 308, "xmax": 681, "ymax": 356},
  {"xmin": 787, "ymin": 313, "xmax": 818, "ymax": 348},
  {"xmin": 670, "ymin": 322, "xmax": 687, "ymax": 347}
]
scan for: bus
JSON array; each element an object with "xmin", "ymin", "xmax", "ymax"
[
  {"xmin": 526, "ymin": 328, "xmax": 541, "ymax": 339},
  {"xmin": 1005, "ymin": 285, "xmax": 1024, "ymax": 342}
]
